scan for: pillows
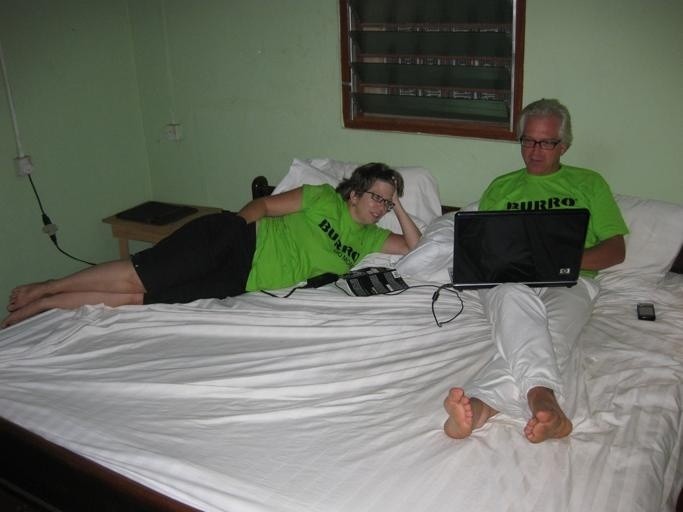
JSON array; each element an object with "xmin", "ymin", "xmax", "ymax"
[{"xmin": 271, "ymin": 159, "xmax": 678, "ymax": 284}]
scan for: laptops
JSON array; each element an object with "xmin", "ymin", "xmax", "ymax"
[{"xmin": 448, "ymin": 208, "xmax": 590, "ymax": 289}]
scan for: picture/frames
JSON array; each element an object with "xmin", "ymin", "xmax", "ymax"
[{"xmin": 339, "ymin": 0, "xmax": 527, "ymax": 145}]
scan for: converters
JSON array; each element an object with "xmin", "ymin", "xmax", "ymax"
[{"xmin": 307, "ymin": 272, "xmax": 338, "ymax": 288}]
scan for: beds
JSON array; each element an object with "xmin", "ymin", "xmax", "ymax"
[{"xmin": 1, "ymin": 173, "xmax": 680, "ymax": 510}]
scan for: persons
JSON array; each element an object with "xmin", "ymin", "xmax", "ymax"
[
  {"xmin": 442, "ymin": 96, "xmax": 630, "ymax": 445},
  {"xmin": 1, "ymin": 162, "xmax": 422, "ymax": 330}
]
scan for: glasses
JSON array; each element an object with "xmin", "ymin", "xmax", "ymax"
[
  {"xmin": 519, "ymin": 134, "xmax": 561, "ymax": 151},
  {"xmin": 365, "ymin": 191, "xmax": 394, "ymax": 209}
]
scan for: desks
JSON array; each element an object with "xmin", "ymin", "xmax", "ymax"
[{"xmin": 100, "ymin": 199, "xmax": 225, "ymax": 260}]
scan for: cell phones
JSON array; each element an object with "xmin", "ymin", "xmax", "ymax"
[{"xmin": 637, "ymin": 303, "xmax": 656, "ymax": 321}]
